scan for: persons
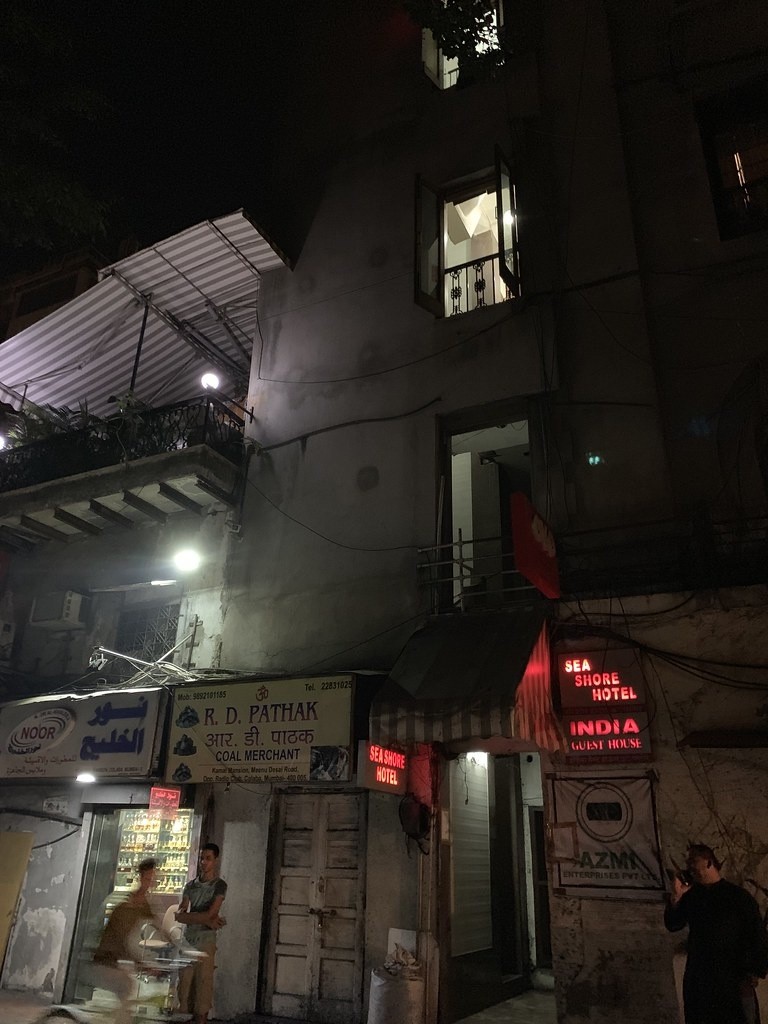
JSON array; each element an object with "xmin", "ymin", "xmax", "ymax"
[
  {"xmin": 664, "ymin": 844, "xmax": 768, "ymax": 1024},
  {"xmin": 88, "ymin": 859, "xmax": 211, "ymax": 1024},
  {"xmin": 173, "ymin": 844, "xmax": 227, "ymax": 1022}
]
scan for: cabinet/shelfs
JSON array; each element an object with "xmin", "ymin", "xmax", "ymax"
[{"xmin": 116, "ymin": 808, "xmax": 193, "ymax": 893}]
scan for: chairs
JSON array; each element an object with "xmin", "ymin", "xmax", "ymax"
[{"xmin": 137, "ymin": 903, "xmax": 185, "ymax": 984}]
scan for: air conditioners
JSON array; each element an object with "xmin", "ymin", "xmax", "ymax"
[{"xmin": 30, "ymin": 590, "xmax": 92, "ymax": 633}]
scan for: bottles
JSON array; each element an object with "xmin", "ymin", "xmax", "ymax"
[
  {"xmin": 678, "ymin": 868, "xmax": 695, "ymax": 886},
  {"xmin": 163, "ymin": 993, "xmax": 175, "ymax": 1015}
]
